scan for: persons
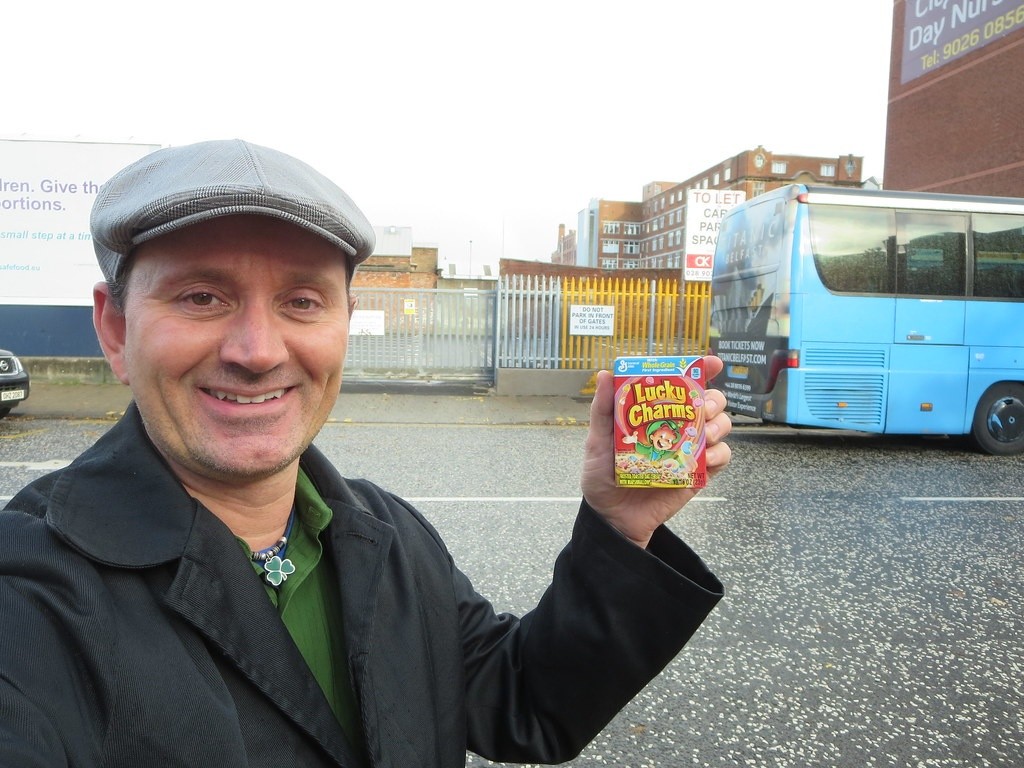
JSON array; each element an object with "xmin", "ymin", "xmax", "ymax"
[{"xmin": 0, "ymin": 138, "xmax": 732, "ymax": 768}]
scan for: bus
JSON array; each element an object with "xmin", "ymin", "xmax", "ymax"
[{"xmin": 703, "ymin": 184, "xmax": 1024, "ymax": 457}]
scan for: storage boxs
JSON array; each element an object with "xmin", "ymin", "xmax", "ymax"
[{"xmin": 614, "ymin": 355, "xmax": 706, "ymax": 488}]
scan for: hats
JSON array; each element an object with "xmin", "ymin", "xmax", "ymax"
[{"xmin": 89, "ymin": 138, "xmax": 376, "ymax": 284}]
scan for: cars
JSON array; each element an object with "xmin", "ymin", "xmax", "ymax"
[{"xmin": 0, "ymin": 348, "xmax": 30, "ymax": 421}]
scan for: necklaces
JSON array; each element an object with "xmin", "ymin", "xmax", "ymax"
[{"xmin": 250, "ymin": 510, "xmax": 295, "ymax": 587}]
